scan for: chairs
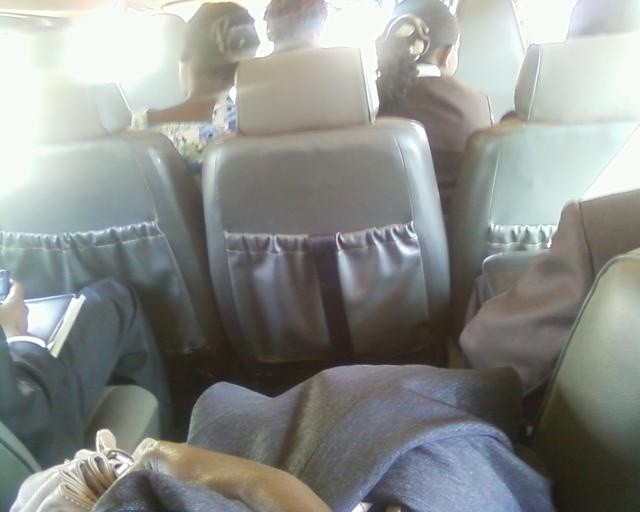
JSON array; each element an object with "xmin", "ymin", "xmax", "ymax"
[
  {"xmin": 448, "ymin": 32, "xmax": 639, "ymax": 346},
  {"xmin": 520, "ymin": 243, "xmax": 639, "ymax": 510},
  {"xmin": 0, "ymin": 382, "xmax": 170, "ymax": 510},
  {"xmin": 113, "ymin": 14, "xmax": 193, "ymax": 124},
  {"xmin": 445, "ymin": 4, "xmax": 533, "ymax": 124},
  {"xmin": 3, "ymin": 76, "xmax": 224, "ymax": 394},
  {"xmin": 560, "ymin": 2, "xmax": 637, "ymax": 42},
  {"xmin": 201, "ymin": 45, "xmax": 450, "ymax": 399},
  {"xmin": 3, "ymin": 12, "xmax": 93, "ymax": 97}
]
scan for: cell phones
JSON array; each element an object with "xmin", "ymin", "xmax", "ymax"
[{"xmin": 0, "ymin": 269, "xmax": 9, "ymax": 302}]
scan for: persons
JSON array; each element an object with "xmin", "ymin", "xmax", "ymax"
[
  {"xmin": 457, "ymin": 188, "xmax": 640, "ymax": 422},
  {"xmin": 130, "ymin": 2, "xmax": 261, "ymax": 194},
  {"xmin": 0, "ymin": 275, "xmax": 176, "ymax": 470},
  {"xmin": 9, "ymin": 364, "xmax": 559, "ymax": 512},
  {"xmin": 375, "ymin": 0, "xmax": 493, "ymax": 229},
  {"xmin": 262, "ymin": 0, "xmax": 329, "ymax": 53},
  {"xmin": 566, "ymin": 0, "xmax": 640, "ymax": 38}
]
